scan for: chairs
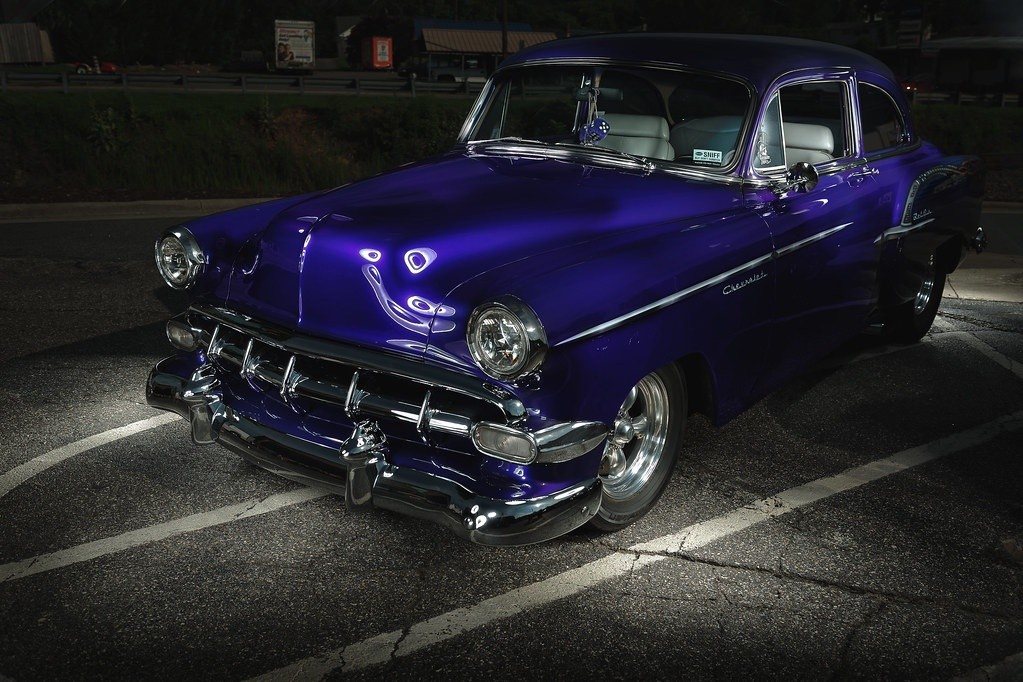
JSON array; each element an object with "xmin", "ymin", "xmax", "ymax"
[
  {"xmin": 733, "ymin": 122, "xmax": 835, "ymax": 170},
  {"xmin": 586, "ymin": 113, "xmax": 675, "ymax": 161}
]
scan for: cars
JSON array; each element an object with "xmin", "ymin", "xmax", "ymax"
[
  {"xmin": 896, "ymin": 7, "xmax": 933, "ymax": 45},
  {"xmin": 73, "ymin": 56, "xmax": 119, "ymax": 75},
  {"xmin": 397, "ymin": 51, "xmax": 501, "ymax": 95},
  {"xmin": 144, "ymin": 32, "xmax": 992, "ymax": 548}
]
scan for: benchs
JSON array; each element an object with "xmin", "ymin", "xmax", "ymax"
[{"xmin": 669, "ymin": 116, "xmax": 843, "ymax": 160}]
